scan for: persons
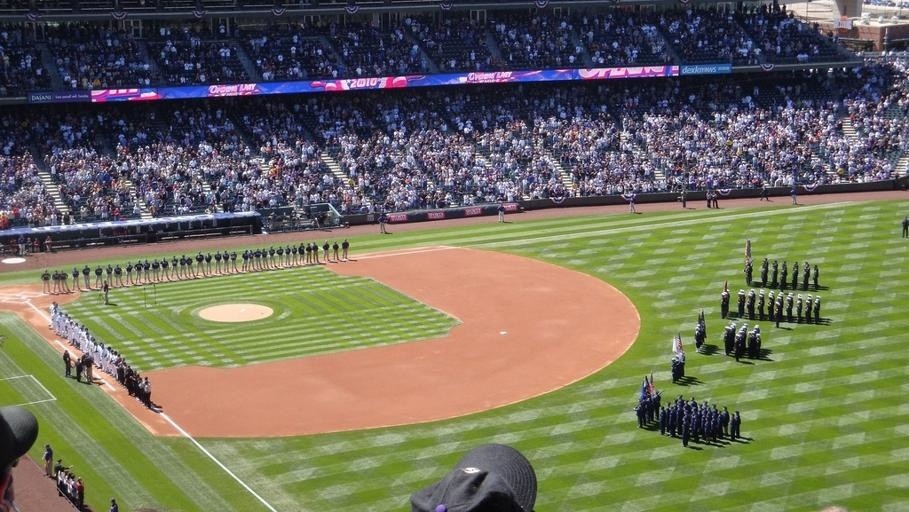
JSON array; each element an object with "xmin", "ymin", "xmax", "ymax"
[
  {"xmin": 0, "ymin": 407, "xmax": 124, "ymax": 511},
  {"xmin": 628, "ymin": 287, "xmax": 823, "ymax": 446},
  {"xmin": 0, "ymin": 0, "xmax": 908, "ymax": 254},
  {"xmin": 42, "ymin": 267, "xmax": 189, "ymax": 408},
  {"xmin": 42, "ymin": 239, "xmax": 349, "ymax": 294}
]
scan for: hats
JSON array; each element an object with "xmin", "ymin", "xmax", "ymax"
[
  {"xmin": 0, "ymin": 405, "xmax": 38, "ymax": 467},
  {"xmin": 695, "ymin": 319, "xmax": 704, "ymax": 336},
  {"xmin": 411, "ymin": 444, "xmax": 537, "ymax": 512},
  {"xmin": 721, "ymin": 289, "xmax": 821, "ymax": 307},
  {"xmin": 724, "ymin": 322, "xmax": 760, "ymax": 338},
  {"xmin": 671, "ymin": 351, "xmax": 683, "ymax": 362}
]
